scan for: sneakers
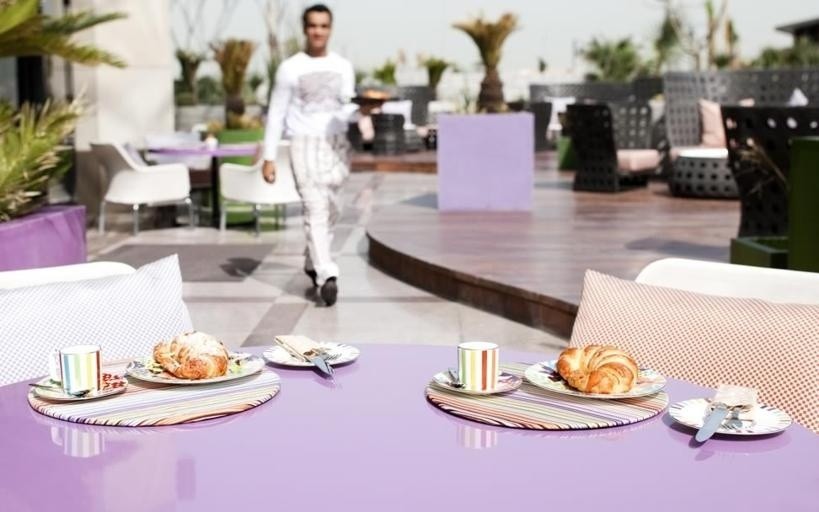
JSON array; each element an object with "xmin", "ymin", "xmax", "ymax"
[
  {"xmin": 321, "ymin": 281, "xmax": 336, "ymax": 305},
  {"xmin": 306, "ymin": 270, "xmax": 317, "ymax": 287}
]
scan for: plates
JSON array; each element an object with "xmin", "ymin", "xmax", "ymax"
[
  {"xmin": 350, "ymin": 96, "xmax": 398, "ymax": 107},
  {"xmin": 263, "ymin": 341, "xmax": 360, "ymax": 368},
  {"xmin": 524, "ymin": 360, "xmax": 669, "ymax": 400},
  {"xmin": 667, "ymin": 396, "xmax": 792, "ymax": 436},
  {"xmin": 431, "ymin": 367, "xmax": 523, "ymax": 396},
  {"xmin": 34, "ymin": 372, "xmax": 129, "ymax": 404},
  {"xmin": 126, "ymin": 350, "xmax": 266, "ymax": 386}
]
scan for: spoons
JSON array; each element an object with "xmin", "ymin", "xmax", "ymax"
[{"xmin": 28, "ymin": 382, "xmax": 93, "ymax": 397}]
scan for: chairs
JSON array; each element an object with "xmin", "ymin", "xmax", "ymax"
[
  {"xmin": 536, "ymin": 97, "xmax": 664, "ymax": 192},
  {"xmin": 218, "ymin": 153, "xmax": 301, "ymax": 233},
  {"xmin": 721, "ymin": 104, "xmax": 819, "ymax": 238},
  {"xmin": 634, "ymin": 258, "xmax": 819, "ymax": 304},
  {"xmin": 370, "ymin": 113, "xmax": 404, "ymax": 155},
  {"xmin": 0, "ymin": 261, "xmax": 137, "ymax": 290},
  {"xmin": 89, "ymin": 142, "xmax": 195, "ymax": 236}
]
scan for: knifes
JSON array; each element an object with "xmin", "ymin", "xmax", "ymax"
[{"xmin": 695, "ymin": 404, "xmax": 728, "ymax": 444}]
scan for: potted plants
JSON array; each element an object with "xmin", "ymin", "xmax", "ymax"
[{"xmin": 0, "ymin": 0, "xmax": 132, "ymax": 270}]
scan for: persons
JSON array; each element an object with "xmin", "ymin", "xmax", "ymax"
[{"xmin": 261, "ymin": 4, "xmax": 385, "ymax": 308}]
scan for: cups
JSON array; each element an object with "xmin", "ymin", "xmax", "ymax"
[
  {"xmin": 456, "ymin": 341, "xmax": 500, "ymax": 391},
  {"xmin": 59, "ymin": 343, "xmax": 104, "ymax": 397}
]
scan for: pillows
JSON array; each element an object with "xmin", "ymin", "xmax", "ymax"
[
  {"xmin": 0, "ymin": 254, "xmax": 194, "ymax": 386},
  {"xmin": 787, "ymin": 88, "xmax": 808, "ymax": 108},
  {"xmin": 357, "ymin": 100, "xmax": 415, "ymax": 135},
  {"xmin": 699, "ymin": 97, "xmax": 754, "ymax": 148},
  {"xmin": 569, "ymin": 270, "xmax": 819, "ymax": 433}
]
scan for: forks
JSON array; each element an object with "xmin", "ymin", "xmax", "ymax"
[{"xmin": 720, "ymin": 405, "xmax": 743, "ymax": 431}]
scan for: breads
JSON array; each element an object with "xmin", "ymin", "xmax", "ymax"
[
  {"xmin": 558, "ymin": 345, "xmax": 639, "ymax": 396},
  {"xmin": 155, "ymin": 330, "xmax": 229, "ymax": 380},
  {"xmin": 360, "ymin": 89, "xmax": 388, "ymax": 100}
]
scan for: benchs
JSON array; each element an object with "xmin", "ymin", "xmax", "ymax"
[
  {"xmin": 664, "ymin": 66, "xmax": 819, "ymax": 174},
  {"xmin": 354, "ymin": 86, "xmax": 428, "ymax": 125},
  {"xmin": 530, "ymin": 79, "xmax": 664, "ymax": 129}
]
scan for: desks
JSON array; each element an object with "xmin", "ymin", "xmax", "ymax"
[{"xmin": 145, "ymin": 144, "xmax": 258, "ymax": 219}]
